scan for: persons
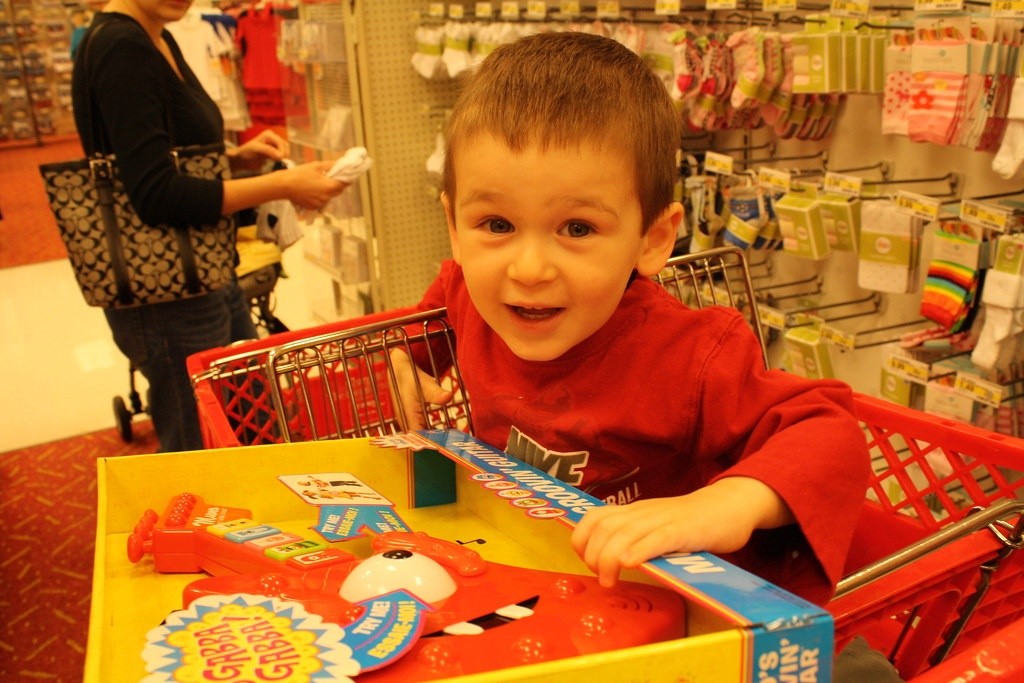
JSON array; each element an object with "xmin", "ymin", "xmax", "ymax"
[
  {"xmin": 388, "ymin": 26, "xmax": 872, "ymax": 608},
  {"xmin": 68, "ymin": 1, "xmax": 355, "ymax": 457}
]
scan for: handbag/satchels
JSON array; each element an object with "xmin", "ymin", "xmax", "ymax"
[{"xmin": 38, "ymin": 22, "xmax": 237, "ymax": 309}]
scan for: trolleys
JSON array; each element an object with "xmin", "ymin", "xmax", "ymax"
[{"xmin": 183, "ymin": 243, "xmax": 1023, "ymax": 683}]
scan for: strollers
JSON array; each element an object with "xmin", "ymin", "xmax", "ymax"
[{"xmin": 112, "ymin": 160, "xmax": 306, "ymax": 443}]
